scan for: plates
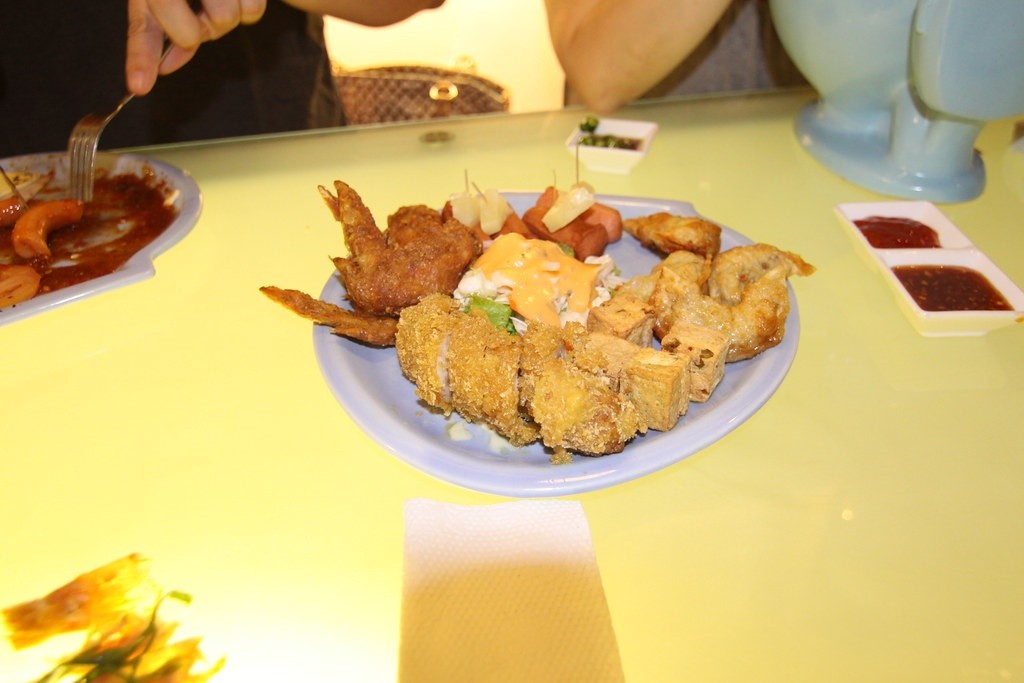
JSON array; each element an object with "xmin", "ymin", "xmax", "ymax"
[
  {"xmin": 0, "ymin": 152, "xmax": 202, "ymax": 326},
  {"xmin": 565, "ymin": 116, "xmax": 658, "ymax": 173},
  {"xmin": 836, "ymin": 200, "xmax": 1024, "ymax": 339},
  {"xmin": 311, "ymin": 193, "xmax": 799, "ymax": 499}
]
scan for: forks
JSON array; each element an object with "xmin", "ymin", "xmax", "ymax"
[{"xmin": 64, "ymin": 1, "xmax": 203, "ymax": 204}]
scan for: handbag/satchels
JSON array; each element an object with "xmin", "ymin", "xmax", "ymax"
[{"xmin": 335, "ymin": 65, "xmax": 509, "ymax": 125}]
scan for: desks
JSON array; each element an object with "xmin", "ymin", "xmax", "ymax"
[{"xmin": 0, "ymin": 86, "xmax": 1024, "ymax": 683}]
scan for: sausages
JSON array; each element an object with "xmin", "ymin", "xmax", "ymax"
[
  {"xmin": 440, "ymin": 187, "xmax": 623, "ymax": 262},
  {"xmin": 0, "ymin": 195, "xmax": 85, "ymax": 261}
]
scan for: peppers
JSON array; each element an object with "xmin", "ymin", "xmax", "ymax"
[{"xmin": 576, "ymin": 117, "xmax": 626, "ymax": 148}]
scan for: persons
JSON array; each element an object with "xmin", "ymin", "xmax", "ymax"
[
  {"xmin": 540, "ymin": 0, "xmax": 813, "ymax": 109},
  {"xmin": 0, "ymin": 0, "xmax": 446, "ymax": 155}
]
